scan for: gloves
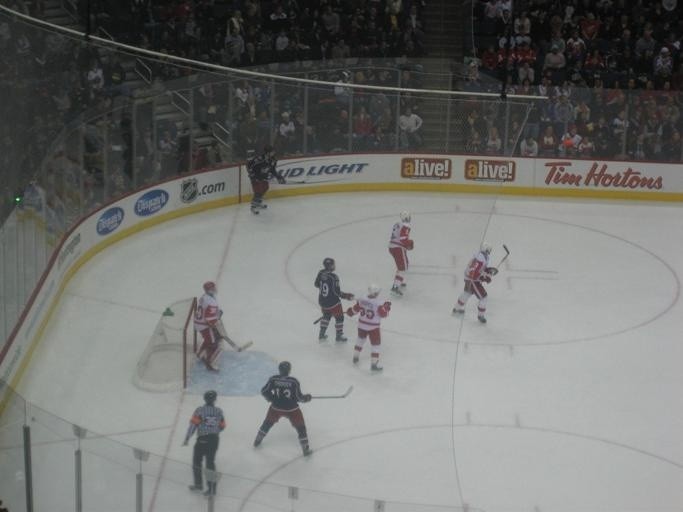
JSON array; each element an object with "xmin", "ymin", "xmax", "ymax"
[
  {"xmin": 485, "ymin": 267, "xmax": 498, "ymax": 275},
  {"xmin": 480, "ymin": 275, "xmax": 491, "ymax": 284},
  {"xmin": 278, "ymin": 176, "xmax": 286, "ymax": 184}
]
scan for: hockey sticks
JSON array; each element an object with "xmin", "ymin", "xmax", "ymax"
[
  {"xmin": 311, "ymin": 386, "xmax": 353, "ymax": 398},
  {"xmin": 223, "ymin": 336, "xmax": 253, "ymax": 352},
  {"xmin": 496, "ymin": 244, "xmax": 509, "ymax": 268},
  {"xmin": 263, "ymin": 175, "xmax": 310, "ymax": 184}
]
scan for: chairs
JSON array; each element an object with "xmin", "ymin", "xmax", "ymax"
[{"xmin": 463, "ymin": 7, "xmax": 682, "ymax": 160}]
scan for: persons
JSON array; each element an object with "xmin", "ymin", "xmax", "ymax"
[
  {"xmin": 388, "ymin": 209, "xmax": 414, "ymax": 296},
  {"xmin": 193, "ymin": 281, "xmax": 228, "ymax": 371},
  {"xmin": 462, "ymin": 0, "xmax": 682, "ymax": 164},
  {"xmin": 1, "ymin": 1, "xmax": 429, "ymax": 162},
  {"xmin": 253, "ymin": 361, "xmax": 312, "ymax": 456},
  {"xmin": 314, "ymin": 257, "xmax": 354, "ymax": 341},
  {"xmin": 1, "ymin": 145, "xmax": 162, "ymax": 227},
  {"xmin": 247, "ymin": 143, "xmax": 285, "ymax": 214},
  {"xmin": 347, "ymin": 284, "xmax": 392, "ymax": 371},
  {"xmin": 452, "ymin": 241, "xmax": 498, "ymax": 323},
  {"xmin": 181, "ymin": 390, "xmax": 226, "ymax": 496}
]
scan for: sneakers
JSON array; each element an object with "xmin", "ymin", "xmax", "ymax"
[{"xmin": 251, "ymin": 203, "xmax": 267, "ymax": 214}]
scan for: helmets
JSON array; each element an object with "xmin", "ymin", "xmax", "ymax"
[
  {"xmin": 400, "ymin": 211, "xmax": 411, "ymax": 222},
  {"xmin": 480, "ymin": 241, "xmax": 492, "ymax": 255},
  {"xmin": 323, "ymin": 258, "xmax": 335, "ymax": 272}
]
